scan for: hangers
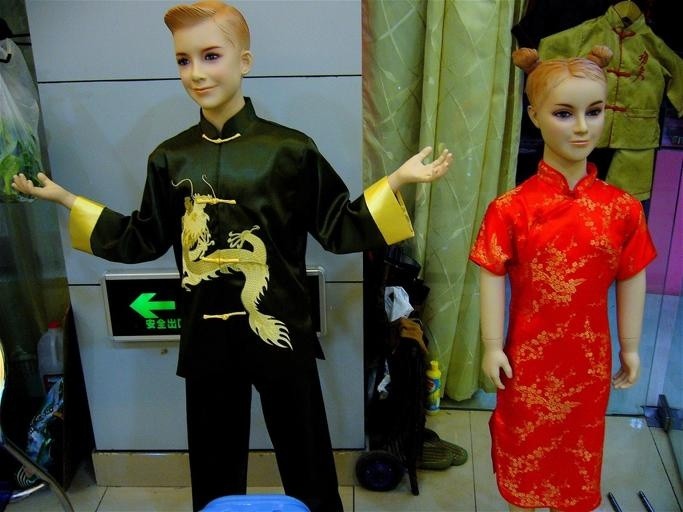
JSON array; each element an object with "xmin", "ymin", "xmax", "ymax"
[{"xmin": 612, "ymin": 0, "xmax": 642, "ymax": 27}]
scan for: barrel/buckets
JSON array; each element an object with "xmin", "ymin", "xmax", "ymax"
[{"xmin": 35, "ymin": 322, "xmax": 66, "ymax": 416}]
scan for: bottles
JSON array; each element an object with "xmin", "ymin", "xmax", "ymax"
[{"xmin": 425, "ymin": 361, "xmax": 440, "ymax": 416}]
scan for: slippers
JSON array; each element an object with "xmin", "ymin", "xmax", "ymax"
[
  {"xmin": 11, "ymin": 466, "xmax": 48, "ymax": 500},
  {"xmin": 417, "ymin": 427, "xmax": 468, "ymax": 470}
]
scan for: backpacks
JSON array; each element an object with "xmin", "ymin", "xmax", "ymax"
[{"xmin": 28, "ymin": 376, "xmax": 68, "ymax": 478}]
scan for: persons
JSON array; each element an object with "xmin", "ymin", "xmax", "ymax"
[
  {"xmin": 11, "ymin": 0, "xmax": 453, "ymax": 511},
  {"xmin": 468, "ymin": 47, "xmax": 657, "ymax": 512}
]
